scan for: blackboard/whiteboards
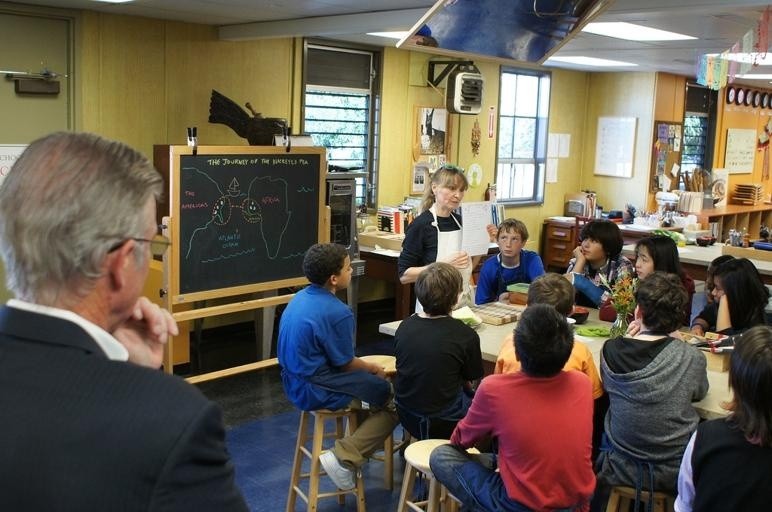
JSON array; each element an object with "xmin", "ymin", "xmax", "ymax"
[{"xmin": 169, "ymin": 146, "xmax": 326, "ymax": 305}]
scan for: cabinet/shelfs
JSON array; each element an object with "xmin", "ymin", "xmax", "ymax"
[{"xmin": 542, "ymin": 218, "xmax": 622, "ymax": 273}]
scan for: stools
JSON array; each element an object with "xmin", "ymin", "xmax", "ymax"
[
  {"xmin": 605, "ymin": 486, "xmax": 669, "ymax": 511},
  {"xmin": 343, "ymin": 356, "xmax": 411, "ymax": 491},
  {"xmin": 286, "ymin": 407, "xmax": 366, "ymax": 512},
  {"xmin": 396, "ymin": 438, "xmax": 480, "ymax": 512}
]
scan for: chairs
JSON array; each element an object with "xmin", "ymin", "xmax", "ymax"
[{"xmin": 569, "ymin": 215, "xmax": 592, "ymax": 259}]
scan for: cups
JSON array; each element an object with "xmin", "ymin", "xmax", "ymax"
[{"xmin": 595, "ymin": 206, "xmax": 603, "ymax": 218}]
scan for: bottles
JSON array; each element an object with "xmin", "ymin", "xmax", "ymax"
[
  {"xmin": 485, "ymin": 183, "xmax": 491, "ymax": 200},
  {"xmin": 743, "ymin": 233, "xmax": 749, "ymax": 248}
]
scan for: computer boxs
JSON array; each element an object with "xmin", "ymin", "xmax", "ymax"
[{"xmin": 326, "ymin": 179, "xmax": 356, "ymax": 261}]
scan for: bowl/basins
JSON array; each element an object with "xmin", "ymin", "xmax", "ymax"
[
  {"xmin": 697, "ymin": 239, "xmax": 710, "ymax": 246},
  {"xmin": 570, "ymin": 306, "xmax": 589, "ymax": 324},
  {"xmin": 567, "ymin": 317, "xmax": 576, "ymax": 324},
  {"xmin": 706, "ymin": 237, "xmax": 717, "ymax": 245}
]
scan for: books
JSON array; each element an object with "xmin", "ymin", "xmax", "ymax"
[
  {"xmin": 460, "ymin": 201, "xmax": 491, "ymax": 256},
  {"xmin": 471, "ymin": 301, "xmax": 524, "ymax": 325},
  {"xmin": 377, "ymin": 198, "xmax": 424, "ymax": 235},
  {"xmin": 564, "ymin": 192, "xmax": 596, "ymax": 219},
  {"xmin": 491, "ymin": 204, "xmax": 505, "ymax": 227}
]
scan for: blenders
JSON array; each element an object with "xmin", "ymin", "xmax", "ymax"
[{"xmin": 655, "ymin": 191, "xmax": 680, "ymax": 227}]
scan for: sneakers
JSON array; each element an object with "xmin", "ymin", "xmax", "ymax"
[{"xmin": 318, "ymin": 450, "xmax": 355, "ymax": 491}]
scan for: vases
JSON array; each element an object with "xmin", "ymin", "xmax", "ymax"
[{"xmin": 610, "ymin": 314, "xmax": 628, "ymax": 337}]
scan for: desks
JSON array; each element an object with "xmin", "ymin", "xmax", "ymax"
[
  {"xmin": 623, "ymin": 240, "xmax": 772, "ymax": 286},
  {"xmin": 679, "ymin": 203, "xmax": 771, "ymax": 245},
  {"xmin": 378, "ymin": 302, "xmax": 734, "ymax": 423},
  {"xmin": 360, "ymin": 245, "xmax": 411, "ymax": 319}
]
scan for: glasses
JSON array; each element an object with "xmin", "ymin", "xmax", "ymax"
[
  {"xmin": 107, "ymin": 234, "xmax": 173, "ymax": 256},
  {"xmin": 443, "ymin": 163, "xmax": 465, "ymax": 173}
]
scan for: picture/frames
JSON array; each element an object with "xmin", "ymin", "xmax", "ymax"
[{"xmin": 408, "ymin": 105, "xmax": 452, "ymax": 196}]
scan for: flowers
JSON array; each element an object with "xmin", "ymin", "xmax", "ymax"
[{"xmin": 598, "ymin": 270, "xmax": 637, "ymax": 335}]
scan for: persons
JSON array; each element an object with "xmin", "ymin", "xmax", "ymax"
[
  {"xmin": 393, "ymin": 261, "xmax": 484, "ymax": 442},
  {"xmin": 475, "ymin": 217, "xmax": 545, "ymax": 305},
  {"xmin": 429, "ymin": 303, "xmax": 599, "ymax": 512},
  {"xmin": 559, "ymin": 219, "xmax": 633, "ymax": 310},
  {"xmin": 671, "ymin": 326, "xmax": 772, "ymax": 512},
  {"xmin": 493, "ymin": 272, "xmax": 604, "ymax": 400},
  {"xmin": 689, "ymin": 254, "xmax": 769, "ymax": 338},
  {"xmin": 0, "ymin": 129, "xmax": 250, "ymax": 512},
  {"xmin": 277, "ymin": 242, "xmax": 400, "ymax": 491},
  {"xmin": 594, "ymin": 272, "xmax": 709, "ymax": 495},
  {"xmin": 397, "ymin": 163, "xmax": 472, "ymax": 314},
  {"xmin": 599, "ymin": 234, "xmax": 696, "ymax": 327}
]
